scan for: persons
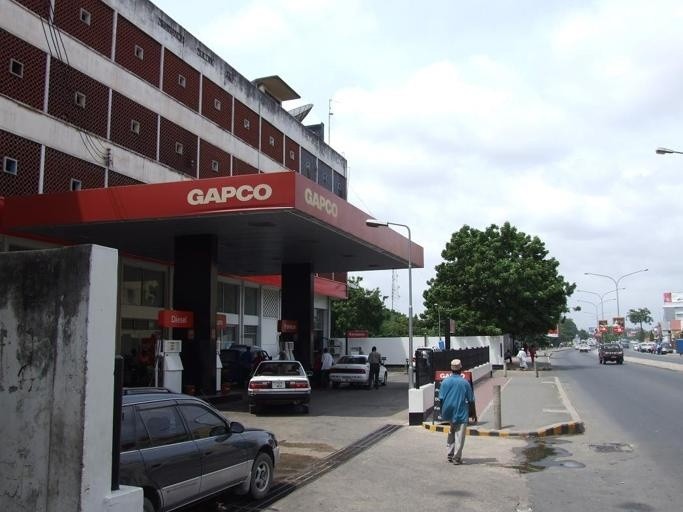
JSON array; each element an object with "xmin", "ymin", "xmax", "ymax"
[
  {"xmin": 320, "ymin": 348, "xmax": 334, "ymax": 386},
  {"xmin": 367, "ymin": 346, "xmax": 383, "ymax": 389},
  {"xmin": 504, "ymin": 342, "xmax": 536, "ymax": 371},
  {"xmin": 647, "ymin": 343, "xmax": 662, "ymax": 355},
  {"xmin": 439, "ymin": 358, "xmax": 476, "ymax": 465},
  {"xmin": 239, "ymin": 345, "xmax": 252, "ymax": 388}
]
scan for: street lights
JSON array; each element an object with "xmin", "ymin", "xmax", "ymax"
[
  {"xmin": 655, "ymin": 145, "xmax": 683, "ymax": 157},
  {"xmin": 366, "ymin": 217, "xmax": 413, "ymax": 388},
  {"xmin": 574, "ymin": 268, "xmax": 654, "ymax": 329}
]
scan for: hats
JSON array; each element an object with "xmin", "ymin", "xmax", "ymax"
[{"xmin": 451, "ymin": 359, "xmax": 462, "ymax": 371}]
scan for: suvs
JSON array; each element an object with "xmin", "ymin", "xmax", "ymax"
[
  {"xmin": 220, "ymin": 346, "xmax": 272, "ymax": 387},
  {"xmin": 115, "ymin": 385, "xmax": 279, "ymax": 511}
]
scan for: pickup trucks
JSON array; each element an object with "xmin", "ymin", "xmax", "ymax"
[{"xmin": 598, "ymin": 342, "xmax": 625, "ymax": 364}]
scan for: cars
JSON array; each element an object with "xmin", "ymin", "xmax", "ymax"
[
  {"xmin": 633, "ymin": 340, "xmax": 672, "ymax": 355},
  {"xmin": 328, "ymin": 353, "xmax": 386, "ymax": 385},
  {"xmin": 578, "ymin": 345, "xmax": 591, "ymax": 353},
  {"xmin": 246, "ymin": 361, "xmax": 312, "ymax": 413}
]
